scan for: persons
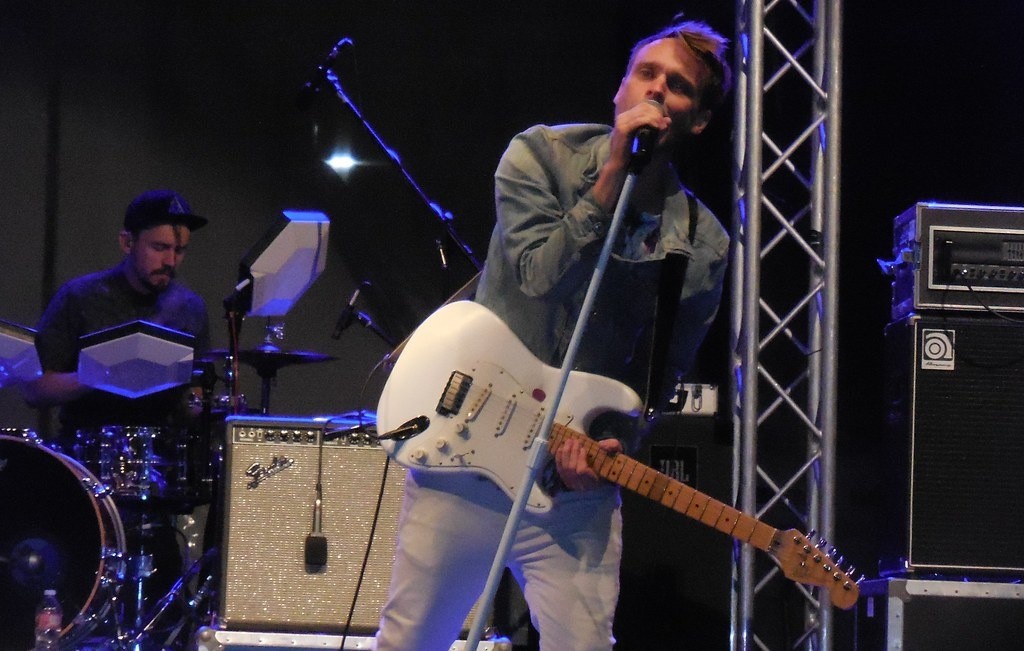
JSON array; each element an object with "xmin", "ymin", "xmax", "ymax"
[
  {"xmin": 17, "ymin": 189, "xmax": 274, "ymax": 649},
  {"xmin": 373, "ymin": 19, "xmax": 732, "ymax": 651}
]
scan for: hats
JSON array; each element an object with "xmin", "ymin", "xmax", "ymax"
[{"xmin": 123, "ymin": 190, "xmax": 210, "ymax": 232}]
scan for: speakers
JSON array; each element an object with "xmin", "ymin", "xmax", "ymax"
[
  {"xmin": 883, "ymin": 313, "xmax": 1024, "ymax": 574},
  {"xmin": 221, "ymin": 414, "xmax": 495, "ymax": 639}
]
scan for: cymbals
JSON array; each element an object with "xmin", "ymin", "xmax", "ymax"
[
  {"xmin": 205, "ymin": 345, "xmax": 345, "ymax": 369},
  {"xmin": 178, "ymin": 404, "xmax": 263, "ymax": 424}
]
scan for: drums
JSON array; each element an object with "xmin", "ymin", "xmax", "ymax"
[
  {"xmin": 74, "ymin": 422, "xmax": 197, "ymax": 516},
  {"xmin": 0, "ymin": 432, "xmax": 131, "ymax": 651}
]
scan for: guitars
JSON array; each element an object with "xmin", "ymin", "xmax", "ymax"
[{"xmin": 374, "ymin": 295, "xmax": 868, "ymax": 611}]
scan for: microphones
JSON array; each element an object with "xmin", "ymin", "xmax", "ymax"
[
  {"xmin": 632, "ymin": 100, "xmax": 664, "ymax": 155},
  {"xmin": 305, "ymin": 495, "xmax": 327, "ymax": 565},
  {"xmin": 331, "ymin": 287, "xmax": 360, "ymax": 340},
  {"xmin": 306, "ymin": 38, "xmax": 353, "ymax": 93}
]
